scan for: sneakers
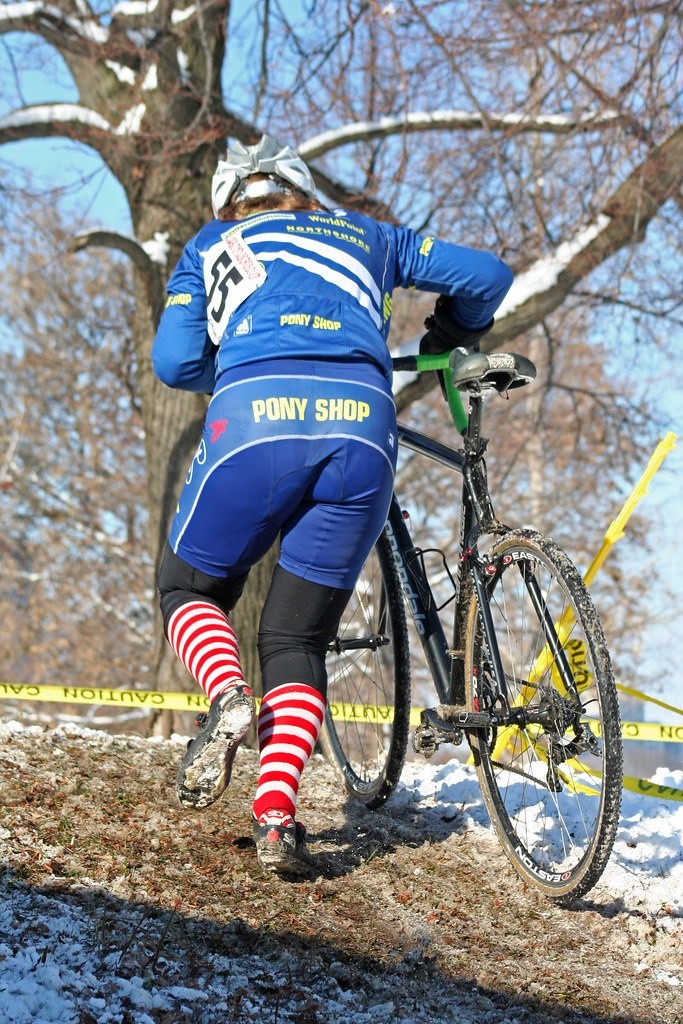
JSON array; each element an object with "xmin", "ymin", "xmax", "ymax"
[
  {"xmin": 176, "ymin": 679, "xmax": 256, "ymax": 812},
  {"xmin": 251, "ymin": 807, "xmax": 313, "ymax": 874}
]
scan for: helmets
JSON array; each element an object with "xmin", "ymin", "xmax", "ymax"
[{"xmin": 211, "ymin": 133, "xmax": 322, "ymax": 220}]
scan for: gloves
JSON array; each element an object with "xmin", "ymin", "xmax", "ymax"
[{"xmin": 419, "ymin": 295, "xmax": 495, "ymax": 369}]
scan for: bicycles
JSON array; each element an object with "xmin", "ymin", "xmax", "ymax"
[{"xmin": 316, "ymin": 345, "xmax": 623, "ymax": 907}]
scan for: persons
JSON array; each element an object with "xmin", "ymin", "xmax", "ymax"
[{"xmin": 151, "ymin": 134, "xmax": 514, "ymax": 876}]
